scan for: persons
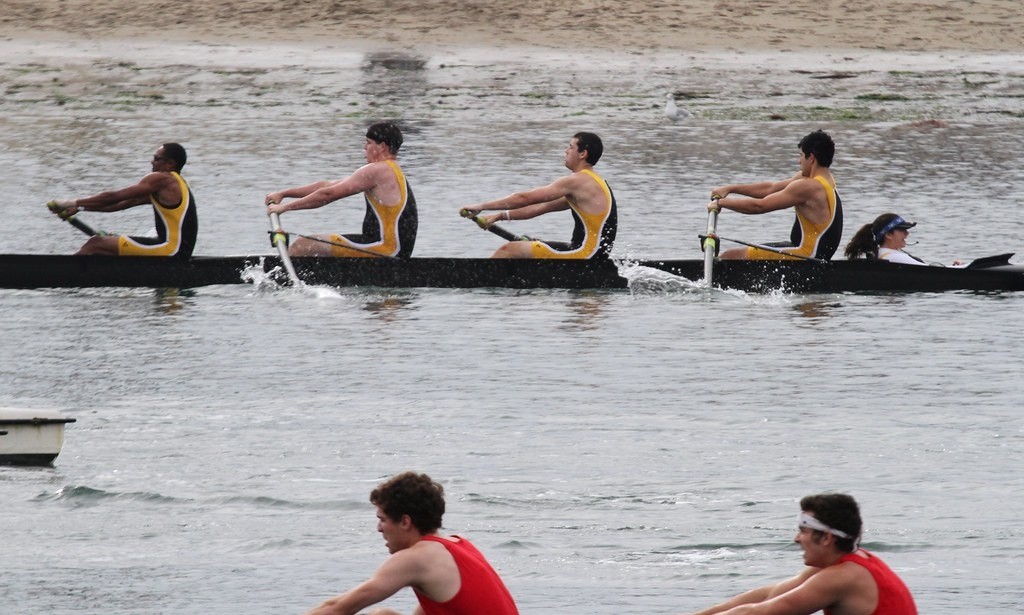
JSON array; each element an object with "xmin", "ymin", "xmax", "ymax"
[
  {"xmin": 303, "ymin": 472, "xmax": 520, "ymax": 615},
  {"xmin": 461, "ymin": 132, "xmax": 618, "ymax": 259},
  {"xmin": 846, "ymin": 214, "xmax": 971, "ymax": 267},
  {"xmin": 47, "ymin": 143, "xmax": 198, "ymax": 257},
  {"xmin": 708, "ymin": 131, "xmax": 843, "ymax": 259},
  {"xmin": 692, "ymin": 494, "xmax": 919, "ymax": 615},
  {"xmin": 265, "ymin": 122, "xmax": 418, "ymax": 257}
]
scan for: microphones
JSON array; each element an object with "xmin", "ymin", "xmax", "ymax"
[{"xmin": 906, "ymin": 241, "xmax": 919, "ymax": 246}]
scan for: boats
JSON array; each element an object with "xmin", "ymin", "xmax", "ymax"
[
  {"xmin": 0, "ymin": 406, "xmax": 77, "ymax": 465},
  {"xmin": 0, "ymin": 251, "xmax": 1024, "ymax": 296}
]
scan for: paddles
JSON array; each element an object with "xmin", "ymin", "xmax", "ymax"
[
  {"xmin": 459, "ymin": 207, "xmax": 546, "ymax": 244},
  {"xmin": 697, "ymin": 193, "xmax": 722, "ymax": 290},
  {"xmin": 46, "ymin": 202, "xmax": 116, "ymax": 237}
]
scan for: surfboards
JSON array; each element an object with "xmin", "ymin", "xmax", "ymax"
[{"xmin": 266, "ymin": 198, "xmax": 302, "ymax": 286}]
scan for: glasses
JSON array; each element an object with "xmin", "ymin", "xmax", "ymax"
[{"xmin": 153, "ymin": 154, "xmax": 169, "ymax": 162}]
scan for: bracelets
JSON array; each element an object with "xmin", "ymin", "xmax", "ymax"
[
  {"xmin": 506, "ymin": 211, "xmax": 510, "ymax": 220},
  {"xmin": 717, "ymin": 199, "xmax": 721, "ymax": 208}
]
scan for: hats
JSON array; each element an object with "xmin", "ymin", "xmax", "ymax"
[{"xmin": 875, "ymin": 215, "xmax": 917, "ymax": 239}]
